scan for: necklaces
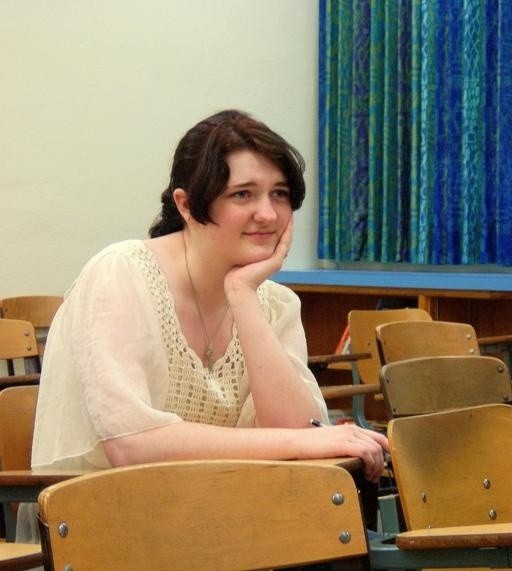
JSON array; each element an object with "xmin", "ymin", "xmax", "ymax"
[{"xmin": 179, "ymin": 229, "xmax": 232, "ymax": 373}]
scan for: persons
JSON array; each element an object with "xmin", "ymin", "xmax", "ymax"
[{"xmin": 13, "ymin": 108, "xmax": 395, "ymax": 571}]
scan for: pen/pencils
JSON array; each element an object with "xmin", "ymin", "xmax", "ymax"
[{"xmin": 310, "ymin": 419, "xmax": 390, "ymax": 457}]
